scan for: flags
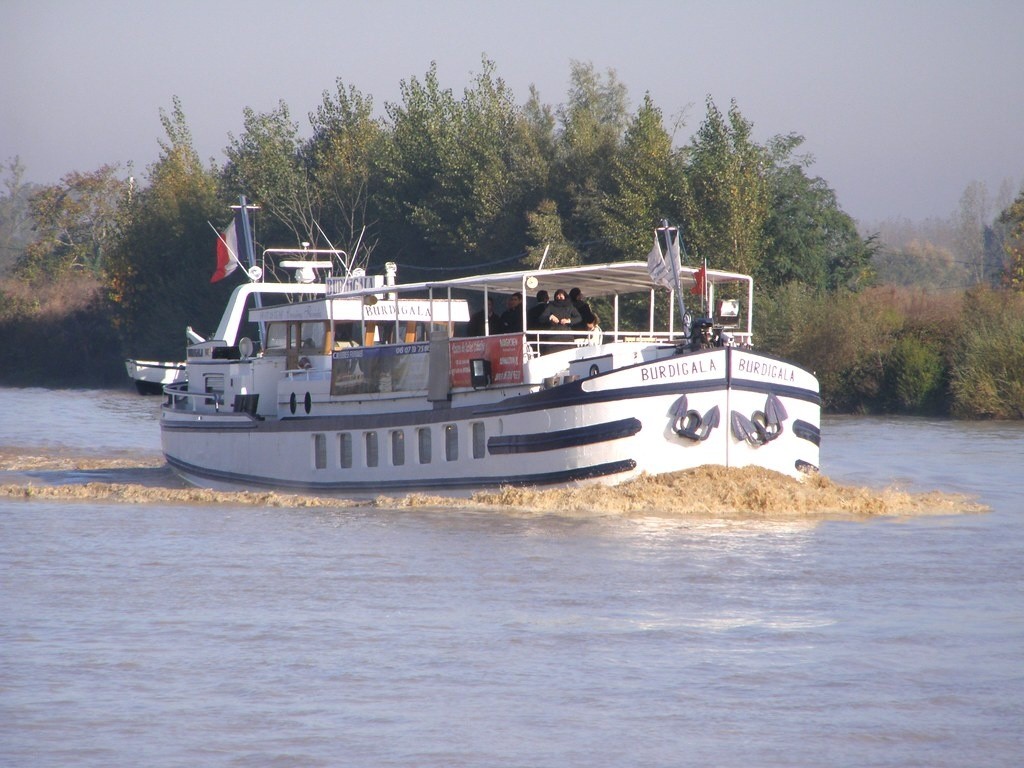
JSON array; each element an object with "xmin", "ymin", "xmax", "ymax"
[
  {"xmin": 689, "ymin": 264, "xmax": 707, "ymax": 296},
  {"xmin": 209, "ymin": 210, "xmax": 251, "ymax": 284},
  {"xmin": 646, "ymin": 230, "xmax": 682, "ymax": 293}
]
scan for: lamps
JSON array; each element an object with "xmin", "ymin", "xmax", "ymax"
[
  {"xmin": 469, "ymin": 358, "xmax": 491, "ymax": 391},
  {"xmin": 717, "ymin": 293, "xmax": 740, "ymax": 318},
  {"xmin": 249, "ymin": 265, "xmax": 262, "ymax": 281}
]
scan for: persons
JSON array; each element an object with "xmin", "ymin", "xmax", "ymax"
[{"xmin": 465, "ymin": 288, "xmax": 603, "ymax": 356}]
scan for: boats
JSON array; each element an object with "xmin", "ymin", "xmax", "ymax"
[
  {"xmin": 124, "ymin": 359, "xmax": 187, "ymax": 395},
  {"xmin": 159, "ymin": 196, "xmax": 820, "ymax": 502}
]
